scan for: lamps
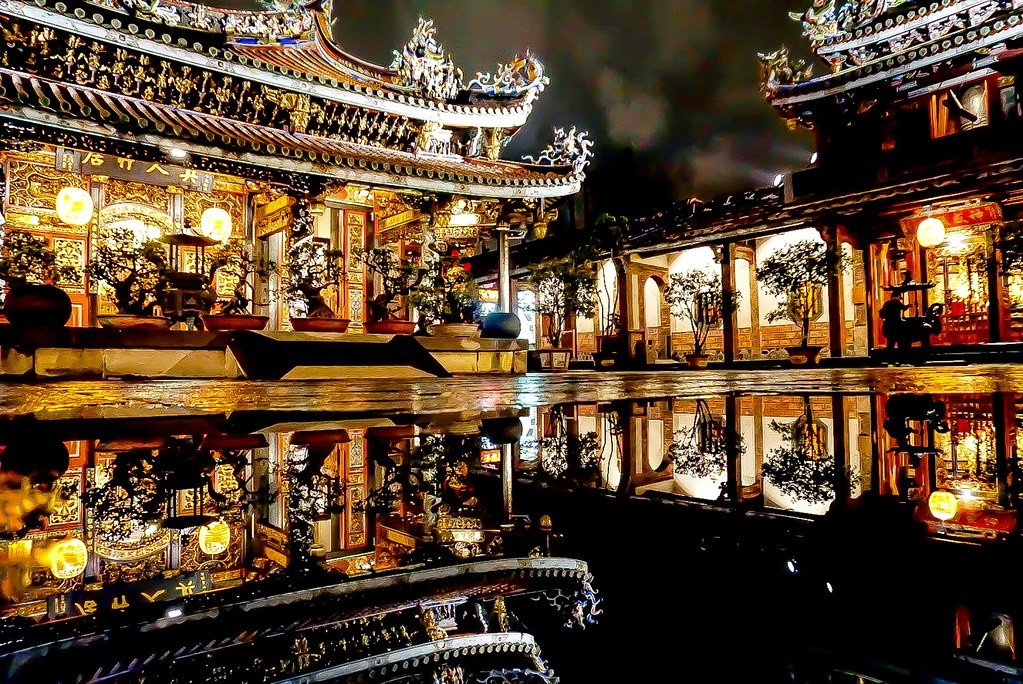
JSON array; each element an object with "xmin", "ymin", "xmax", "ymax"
[
  {"xmin": 929, "ymin": 490, "xmax": 958, "ymax": 537},
  {"xmin": 917, "ymin": 209, "xmax": 946, "ymax": 251}
]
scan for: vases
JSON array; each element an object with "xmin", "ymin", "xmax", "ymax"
[
  {"xmin": 96, "ymin": 314, "xmax": 176, "ymax": 333},
  {"xmin": 362, "ymin": 319, "xmax": 417, "ymax": 335},
  {"xmin": 483, "ymin": 313, "xmax": 522, "ymax": 339},
  {"xmin": 198, "ymin": 313, "xmax": 270, "ymax": 331},
  {"xmin": 4, "ymin": 282, "xmax": 72, "ymax": 328},
  {"xmin": 289, "ymin": 318, "xmax": 351, "ymax": 333},
  {"xmin": 431, "ymin": 322, "xmax": 480, "ymax": 337}
]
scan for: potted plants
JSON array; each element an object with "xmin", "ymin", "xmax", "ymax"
[
  {"xmin": 0, "ymin": 229, "xmax": 81, "ymax": 284},
  {"xmin": 517, "ymin": 214, "xmax": 633, "ymax": 372},
  {"xmin": 277, "ymin": 232, "xmax": 345, "ymax": 301},
  {"xmin": 411, "ymin": 240, "xmax": 480, "ymax": 309},
  {"xmin": 205, "ymin": 238, "xmax": 278, "ymax": 280},
  {"xmin": 663, "ymin": 268, "xmax": 742, "ymax": 370},
  {"xmin": 353, "ymin": 240, "xmax": 437, "ymax": 303},
  {"xmin": 3, "ymin": 396, "xmax": 857, "ymax": 554},
  {"xmin": 85, "ymin": 219, "xmax": 173, "ymax": 305},
  {"xmin": 754, "ymin": 241, "xmax": 855, "ymax": 369}
]
